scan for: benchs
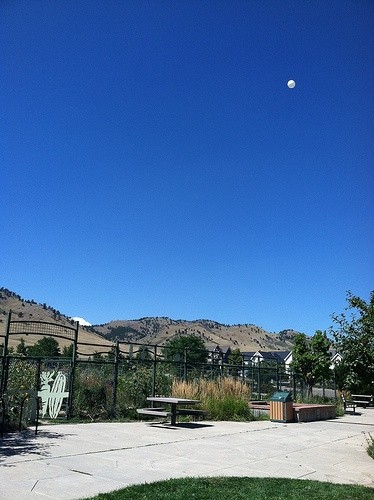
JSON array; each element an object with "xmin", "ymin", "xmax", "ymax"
[
  {"xmin": 174, "ymin": 409, "xmax": 206, "ymax": 421},
  {"xmin": 340, "ymin": 394, "xmax": 356, "ymax": 415},
  {"xmin": 141, "ymin": 406, "xmax": 166, "ymax": 422},
  {"xmin": 137, "ymin": 409, "xmax": 170, "ymax": 423},
  {"xmin": 351, "ymin": 400, "xmax": 369, "ymax": 408},
  {"xmin": 247, "ymin": 400, "xmax": 338, "ymax": 422}
]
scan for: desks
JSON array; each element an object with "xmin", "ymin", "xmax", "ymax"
[
  {"xmin": 146, "ymin": 396, "xmax": 201, "ymax": 426},
  {"xmin": 352, "ymin": 394, "xmax": 374, "ymax": 406}
]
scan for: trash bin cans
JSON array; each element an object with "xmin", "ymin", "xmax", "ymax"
[{"xmin": 270, "ymin": 391, "xmax": 294, "ymax": 423}]
